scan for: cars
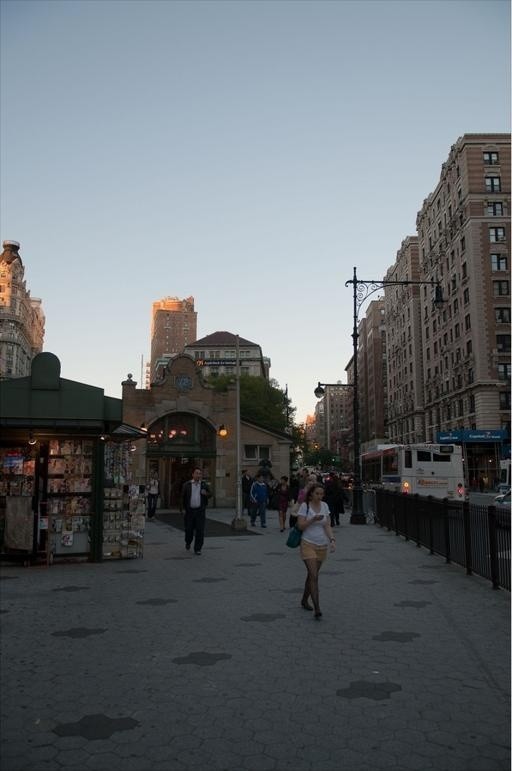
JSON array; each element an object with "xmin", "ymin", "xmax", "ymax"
[
  {"xmin": 302, "ymin": 463, "xmax": 354, "ymax": 485},
  {"xmin": 493, "ymin": 488, "xmax": 511, "ymax": 505},
  {"xmin": 296, "ymin": 460, "xmax": 298, "ymax": 465}
]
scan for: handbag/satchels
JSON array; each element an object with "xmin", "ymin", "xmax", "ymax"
[{"xmin": 286, "ymin": 500, "xmax": 310, "ymax": 549}]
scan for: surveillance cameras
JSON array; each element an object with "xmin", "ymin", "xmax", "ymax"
[{"xmin": 315, "ymin": 388, "xmax": 325, "ymax": 397}]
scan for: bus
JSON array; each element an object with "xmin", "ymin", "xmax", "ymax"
[
  {"xmin": 359, "ymin": 441, "xmax": 466, "ymax": 509},
  {"xmin": 497, "ymin": 458, "xmax": 511, "ymax": 494}
]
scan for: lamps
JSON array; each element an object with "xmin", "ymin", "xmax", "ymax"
[
  {"xmin": 218, "ymin": 422, "xmax": 228, "ymax": 436},
  {"xmin": 27, "ymin": 430, "xmax": 37, "ymax": 444}
]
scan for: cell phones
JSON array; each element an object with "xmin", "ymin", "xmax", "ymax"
[{"xmin": 319, "ymin": 514, "xmax": 324, "ymax": 521}]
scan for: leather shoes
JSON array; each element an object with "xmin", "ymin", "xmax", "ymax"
[
  {"xmin": 314, "ymin": 612, "xmax": 322, "ymax": 618},
  {"xmin": 185, "ymin": 542, "xmax": 190, "ymax": 549},
  {"xmin": 301, "ymin": 600, "xmax": 313, "ymax": 611},
  {"xmin": 195, "ymin": 549, "xmax": 202, "ymax": 555}
]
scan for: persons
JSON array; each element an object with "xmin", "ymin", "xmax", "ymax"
[
  {"xmin": 240, "ymin": 468, "xmax": 354, "ymax": 531},
  {"xmin": 295, "ymin": 485, "xmax": 336, "ymax": 618},
  {"xmin": 479, "ymin": 476, "xmax": 485, "ymax": 493},
  {"xmin": 171, "ymin": 471, "xmax": 186, "ymax": 516},
  {"xmin": 145, "ymin": 471, "xmax": 161, "ymax": 522},
  {"xmin": 180, "ymin": 467, "xmax": 213, "ymax": 556},
  {"xmin": 456, "ymin": 482, "xmax": 464, "ymax": 497}
]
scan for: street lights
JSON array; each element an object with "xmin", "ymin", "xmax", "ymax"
[{"xmin": 314, "ymin": 265, "xmax": 447, "ymax": 528}]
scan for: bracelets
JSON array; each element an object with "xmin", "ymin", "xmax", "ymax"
[{"xmin": 329, "ymin": 536, "xmax": 336, "ymax": 543}]
